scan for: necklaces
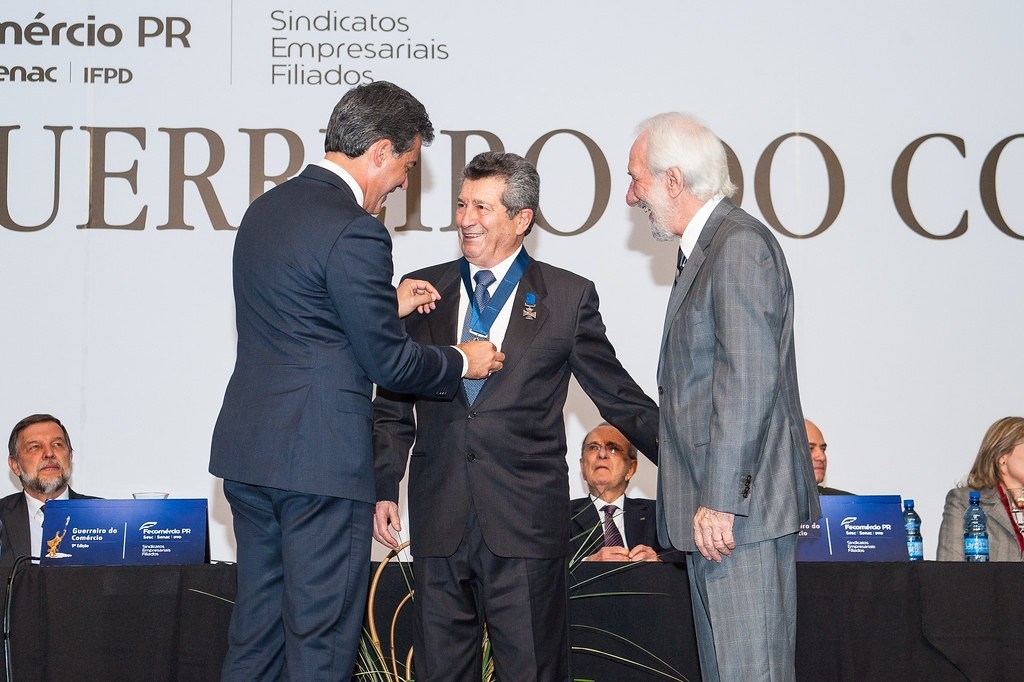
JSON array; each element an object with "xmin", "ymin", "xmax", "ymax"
[{"xmin": 1015, "ymin": 493, "xmax": 1024, "ymax": 502}]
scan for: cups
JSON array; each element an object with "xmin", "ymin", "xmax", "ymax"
[{"xmin": 133, "ymin": 492, "xmax": 169, "ymax": 499}]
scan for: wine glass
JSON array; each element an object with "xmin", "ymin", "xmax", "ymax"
[{"xmin": 1006, "ymin": 488, "xmax": 1024, "ymax": 538}]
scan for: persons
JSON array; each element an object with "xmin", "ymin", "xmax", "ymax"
[
  {"xmin": 805, "ymin": 419, "xmax": 855, "ymax": 494},
  {"xmin": 937, "ymin": 417, "xmax": 1024, "ymax": 562},
  {"xmin": 0, "ymin": 412, "xmax": 108, "ymax": 565},
  {"xmin": 373, "ymin": 153, "xmax": 666, "ymax": 682},
  {"xmin": 565, "ymin": 422, "xmax": 671, "ymax": 561},
  {"xmin": 208, "ymin": 80, "xmax": 504, "ymax": 682},
  {"xmin": 626, "ymin": 113, "xmax": 826, "ymax": 682}
]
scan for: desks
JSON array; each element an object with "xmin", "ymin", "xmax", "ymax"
[{"xmin": 0, "ymin": 562, "xmax": 1024, "ymax": 682}]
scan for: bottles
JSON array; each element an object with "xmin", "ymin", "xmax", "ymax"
[
  {"xmin": 962, "ymin": 491, "xmax": 988, "ymax": 562},
  {"xmin": 902, "ymin": 500, "xmax": 923, "ymax": 562}
]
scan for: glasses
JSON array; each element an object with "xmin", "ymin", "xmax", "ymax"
[{"xmin": 582, "ymin": 442, "xmax": 637, "ymax": 462}]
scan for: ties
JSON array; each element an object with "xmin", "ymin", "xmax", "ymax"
[
  {"xmin": 40, "ymin": 505, "xmax": 45, "ymax": 527},
  {"xmin": 599, "ymin": 504, "xmax": 625, "ymax": 549},
  {"xmin": 460, "ymin": 271, "xmax": 497, "ymax": 406},
  {"xmin": 675, "ymin": 245, "xmax": 687, "ymax": 288}
]
